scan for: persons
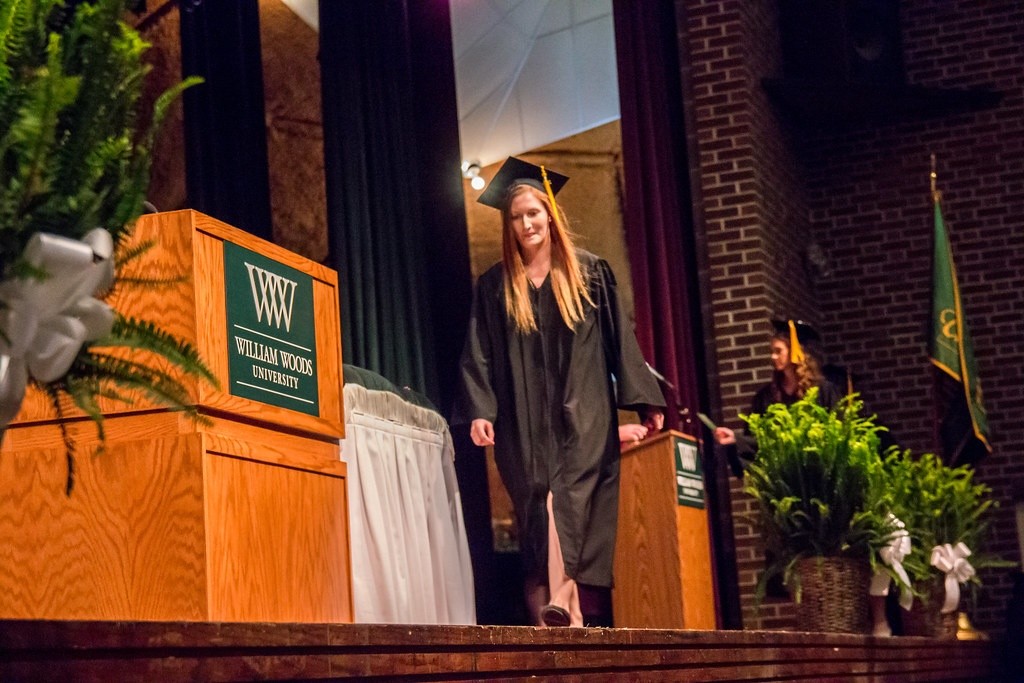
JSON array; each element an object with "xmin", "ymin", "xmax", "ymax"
[
  {"xmin": 453, "ymin": 157, "xmax": 666, "ymax": 627},
  {"xmin": 712, "ymin": 316, "xmax": 844, "ymax": 476}
]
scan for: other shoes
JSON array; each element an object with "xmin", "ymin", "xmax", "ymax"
[{"xmin": 541, "ymin": 605, "xmax": 571, "ymax": 627}]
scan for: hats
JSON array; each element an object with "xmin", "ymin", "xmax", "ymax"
[
  {"xmin": 476, "ymin": 156, "xmax": 570, "ymax": 224},
  {"xmin": 770, "ymin": 317, "xmax": 812, "ymax": 366}
]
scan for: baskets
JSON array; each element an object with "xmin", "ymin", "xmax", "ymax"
[
  {"xmin": 898, "ymin": 571, "xmax": 959, "ymax": 640},
  {"xmin": 789, "ymin": 556, "xmax": 873, "ymax": 633}
]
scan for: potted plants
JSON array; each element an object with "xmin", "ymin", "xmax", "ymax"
[{"xmin": 736, "ymin": 385, "xmax": 1002, "ymax": 642}]
